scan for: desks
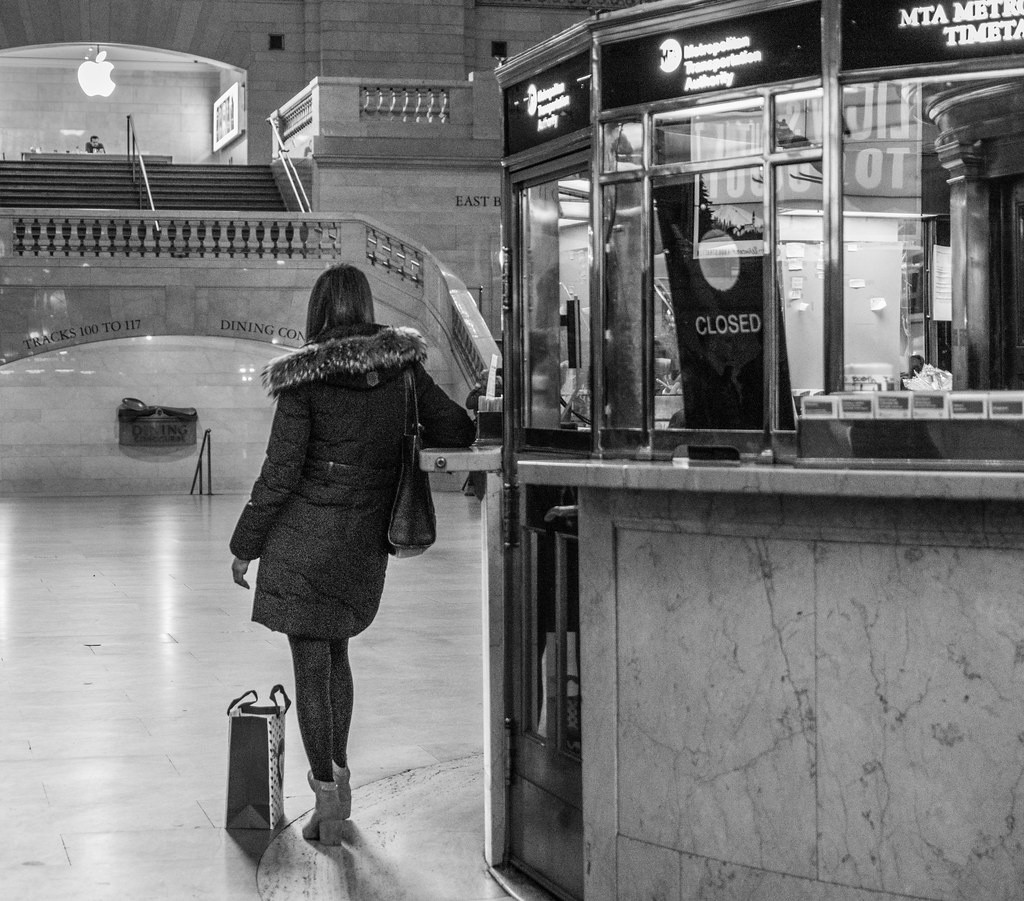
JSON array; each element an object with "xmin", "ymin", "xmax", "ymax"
[{"xmin": 21, "ymin": 151, "xmax": 172, "ymax": 164}]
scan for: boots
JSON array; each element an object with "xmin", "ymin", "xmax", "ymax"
[
  {"xmin": 307, "ymin": 757, "xmax": 352, "ymax": 820},
  {"xmin": 301, "ymin": 776, "xmax": 343, "ymax": 847}
]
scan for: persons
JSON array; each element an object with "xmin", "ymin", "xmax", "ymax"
[
  {"xmin": 228, "ymin": 263, "xmax": 478, "ymax": 848},
  {"xmin": 85, "ymin": 135, "xmax": 105, "ymax": 154}
]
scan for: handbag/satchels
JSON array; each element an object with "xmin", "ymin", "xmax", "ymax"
[
  {"xmin": 386, "ymin": 368, "xmax": 437, "ymax": 560},
  {"xmin": 224, "ymin": 684, "xmax": 292, "ymax": 830}
]
